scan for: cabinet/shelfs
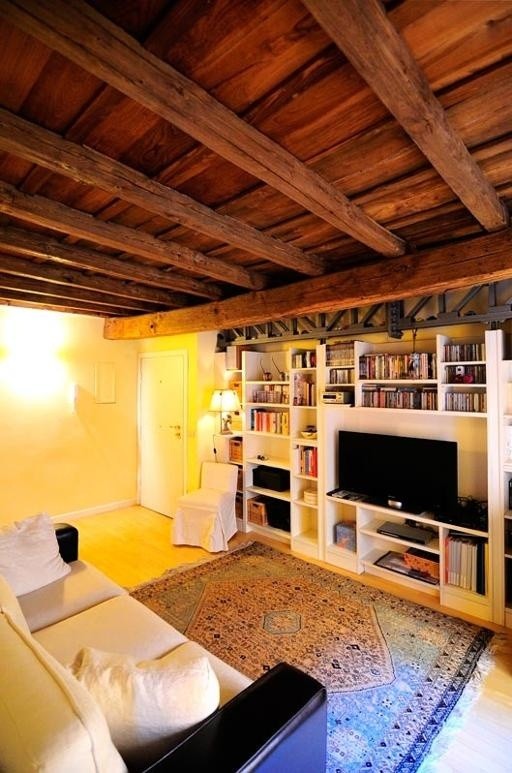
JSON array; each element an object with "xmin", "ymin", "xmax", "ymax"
[
  {"xmin": 289, "ymin": 345, "xmax": 322, "ymax": 554},
  {"xmin": 221, "ymin": 355, "xmax": 243, "ymax": 519},
  {"xmin": 326, "ymin": 409, "xmax": 489, "ymax": 535},
  {"xmin": 323, "ymin": 334, "xmax": 485, "ymax": 412},
  {"xmin": 501, "ymin": 329, "xmax": 512, "ymax": 625},
  {"xmin": 323, "ymin": 499, "xmax": 494, "ymax": 619},
  {"xmin": 242, "ymin": 349, "xmax": 288, "ymax": 549}
]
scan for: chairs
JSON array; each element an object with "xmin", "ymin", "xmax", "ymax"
[{"xmin": 173, "ymin": 461, "xmax": 238, "ymax": 555}]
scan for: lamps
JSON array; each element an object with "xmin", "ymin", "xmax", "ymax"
[{"xmin": 207, "ymin": 389, "xmax": 242, "ymax": 435}]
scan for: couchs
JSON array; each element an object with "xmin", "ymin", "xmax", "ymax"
[{"xmin": 0, "ymin": 523, "xmax": 328, "ymax": 773}]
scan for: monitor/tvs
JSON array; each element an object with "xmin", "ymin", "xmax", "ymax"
[{"xmin": 338, "ymin": 429, "xmax": 458, "ymax": 521}]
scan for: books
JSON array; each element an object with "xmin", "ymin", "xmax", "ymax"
[
  {"xmin": 441, "ymin": 343, "xmax": 486, "ymax": 412},
  {"xmin": 292, "ymin": 351, "xmax": 314, "ymax": 368},
  {"xmin": 253, "ymin": 381, "xmax": 317, "ymax": 407},
  {"xmin": 329, "ymin": 369, "xmax": 355, "ymax": 384},
  {"xmin": 292, "ymin": 447, "xmax": 317, "ymax": 477},
  {"xmin": 359, "ymin": 353, "xmax": 437, "ymax": 379},
  {"xmin": 326, "ymin": 343, "xmax": 354, "ymax": 366},
  {"xmin": 445, "ymin": 537, "xmax": 488, "ymax": 596},
  {"xmin": 362, "ymin": 383, "xmax": 438, "ymax": 410},
  {"xmin": 251, "ymin": 409, "xmax": 289, "ymax": 436}
]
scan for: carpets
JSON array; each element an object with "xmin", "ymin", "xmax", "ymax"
[{"xmin": 124, "ymin": 538, "xmax": 511, "ymax": 773}]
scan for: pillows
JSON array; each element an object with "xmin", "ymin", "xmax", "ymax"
[
  {"xmin": 0, "ymin": 512, "xmax": 72, "ymax": 599},
  {"xmin": 71, "ymin": 640, "xmax": 222, "ymax": 762}
]
